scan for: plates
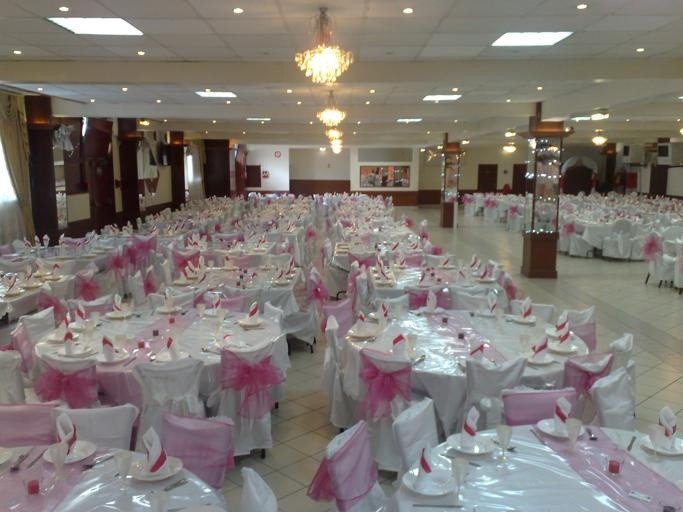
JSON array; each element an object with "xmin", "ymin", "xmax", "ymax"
[
  {"xmin": 47, "ymin": 305, "xmax": 266, "ymax": 365},
  {"xmin": 402, "ymin": 416, "xmax": 683, "ymax": 496},
  {"xmin": 42, "ymin": 440, "xmax": 98, "ymax": 464},
  {"xmin": 130, "ymin": 454, "xmax": 185, "ymax": 481},
  {"xmin": 347, "ymin": 305, "xmax": 578, "ymax": 369},
  {"xmin": 3, "ymin": 271, "xmax": 64, "ymax": 296},
  {"xmin": 0, "ymin": 449, "xmax": 12, "ymax": 466}
]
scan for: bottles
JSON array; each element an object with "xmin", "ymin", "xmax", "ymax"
[{"xmin": 23, "ymin": 479, "xmax": 47, "ymax": 512}]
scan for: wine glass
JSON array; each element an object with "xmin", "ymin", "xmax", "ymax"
[{"xmin": 114, "ymin": 451, "xmax": 132, "ymax": 492}]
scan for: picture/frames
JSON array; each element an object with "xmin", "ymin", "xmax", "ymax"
[{"xmin": 360, "ymin": 166, "xmax": 410, "ymax": 187}]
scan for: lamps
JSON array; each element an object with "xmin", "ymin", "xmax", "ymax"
[
  {"xmin": 316, "ymin": 90, "xmax": 347, "ymax": 127},
  {"xmin": 293, "ymin": 5, "xmax": 355, "ymax": 86}
]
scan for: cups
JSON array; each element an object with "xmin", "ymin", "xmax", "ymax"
[{"xmin": 150, "ymin": 491, "xmax": 171, "ymax": 512}]
